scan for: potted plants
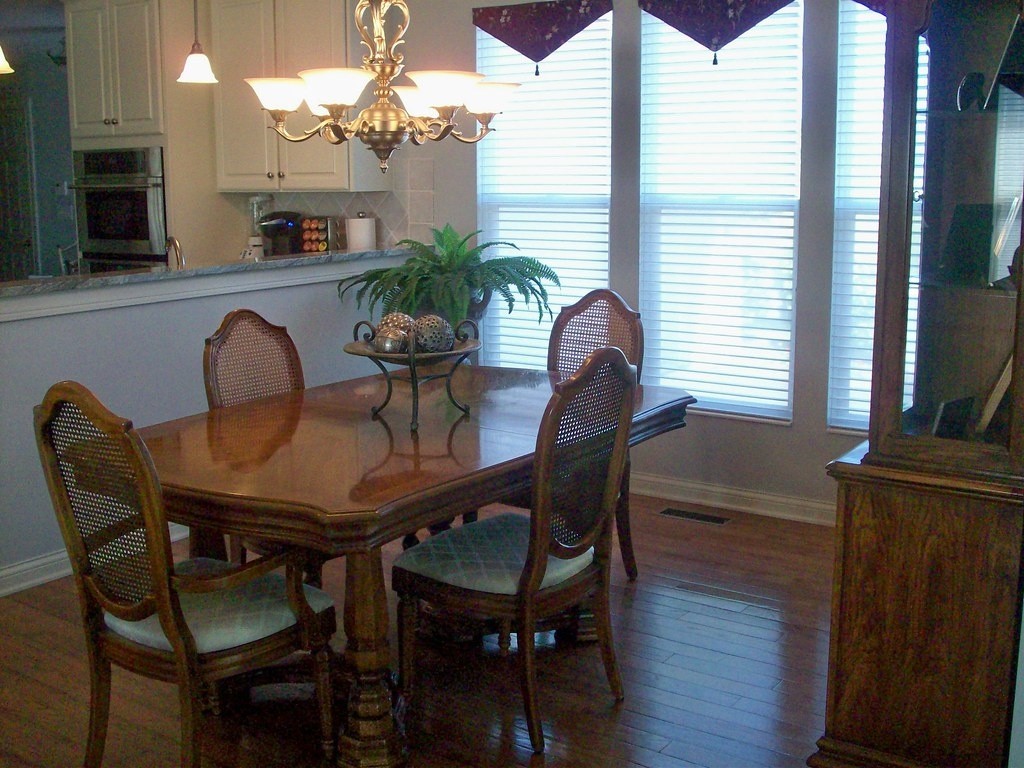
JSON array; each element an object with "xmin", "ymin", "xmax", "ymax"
[{"xmin": 337, "ymin": 224, "xmax": 560, "ymax": 329}]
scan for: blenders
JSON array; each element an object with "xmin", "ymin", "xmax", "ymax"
[{"xmin": 239, "ymin": 195, "xmax": 274, "ymax": 259}]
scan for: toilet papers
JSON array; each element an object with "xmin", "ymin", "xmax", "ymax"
[{"xmin": 344, "ymin": 217, "xmax": 377, "ymax": 250}]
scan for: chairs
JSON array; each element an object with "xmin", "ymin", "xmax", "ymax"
[
  {"xmin": 463, "ymin": 289, "xmax": 646, "ymax": 580},
  {"xmin": 202, "ymin": 308, "xmax": 419, "ymax": 716},
  {"xmin": 391, "ymin": 347, "xmax": 638, "ymax": 754},
  {"xmin": 33, "ymin": 380, "xmax": 340, "ymax": 768}
]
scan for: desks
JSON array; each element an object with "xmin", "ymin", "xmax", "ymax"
[{"xmin": 59, "ymin": 361, "xmax": 699, "ymax": 768}]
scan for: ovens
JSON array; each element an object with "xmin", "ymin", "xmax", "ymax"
[{"xmin": 69, "ymin": 146, "xmax": 167, "ymax": 275}]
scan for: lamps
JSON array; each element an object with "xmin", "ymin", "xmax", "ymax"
[
  {"xmin": 176, "ymin": 0, "xmax": 219, "ymax": 84},
  {"xmin": 243, "ymin": 0, "xmax": 522, "ymax": 174}
]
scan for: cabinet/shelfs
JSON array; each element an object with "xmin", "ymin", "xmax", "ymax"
[
  {"xmin": 789, "ymin": 0, "xmax": 1024, "ymax": 768},
  {"xmin": 63, "ymin": 0, "xmax": 477, "ymax": 265}
]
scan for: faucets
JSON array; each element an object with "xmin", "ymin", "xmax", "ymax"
[{"xmin": 164, "ymin": 236, "xmax": 186, "ymax": 265}]
